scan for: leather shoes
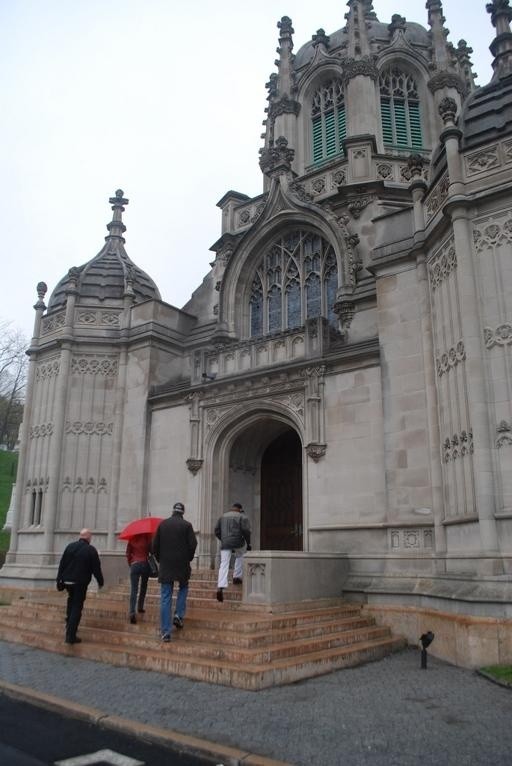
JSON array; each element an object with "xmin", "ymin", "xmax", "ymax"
[{"xmin": 130, "ymin": 609, "xmax": 145, "ymax": 624}]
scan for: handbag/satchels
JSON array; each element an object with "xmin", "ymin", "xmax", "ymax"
[
  {"xmin": 147, "ymin": 555, "xmax": 159, "ymax": 579},
  {"xmin": 56, "ymin": 576, "xmax": 66, "ymax": 592}
]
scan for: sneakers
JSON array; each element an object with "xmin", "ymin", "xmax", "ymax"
[
  {"xmin": 158, "ymin": 633, "xmax": 172, "ymax": 643},
  {"xmin": 217, "ymin": 589, "xmax": 223, "ymax": 602},
  {"xmin": 233, "ymin": 578, "xmax": 242, "ymax": 585},
  {"xmin": 172, "ymin": 614, "xmax": 184, "ymax": 629},
  {"xmin": 64, "ymin": 634, "xmax": 82, "ymax": 645}
]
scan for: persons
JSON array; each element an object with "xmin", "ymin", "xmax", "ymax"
[
  {"xmin": 213, "ymin": 502, "xmax": 252, "ymax": 603},
  {"xmin": 123, "ymin": 532, "xmax": 153, "ymax": 624},
  {"xmin": 148, "ymin": 501, "xmax": 199, "ymax": 642},
  {"xmin": 53, "ymin": 527, "xmax": 105, "ymax": 646}
]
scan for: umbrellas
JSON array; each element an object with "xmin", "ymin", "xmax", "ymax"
[{"xmin": 115, "ymin": 515, "xmax": 167, "ymax": 541}]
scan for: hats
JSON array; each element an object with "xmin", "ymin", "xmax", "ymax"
[
  {"xmin": 172, "ymin": 503, "xmax": 186, "ymax": 514},
  {"xmin": 233, "ymin": 503, "xmax": 245, "ymax": 513}
]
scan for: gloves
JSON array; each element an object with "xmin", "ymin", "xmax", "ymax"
[{"xmin": 246, "ymin": 544, "xmax": 252, "ymax": 551}]
